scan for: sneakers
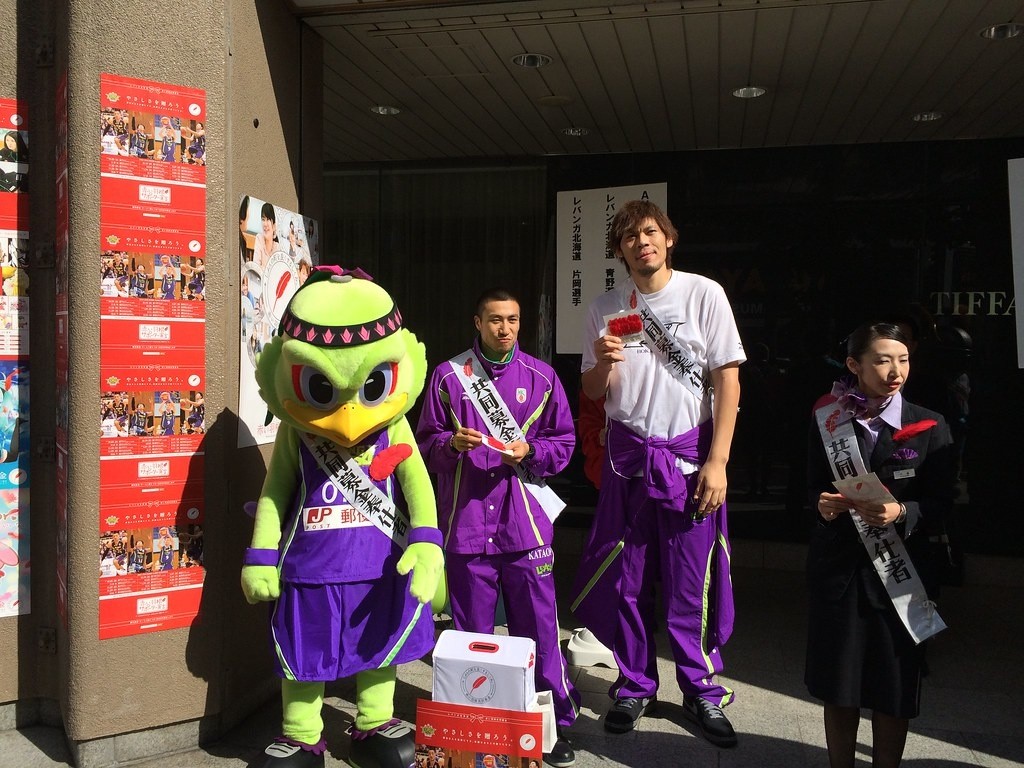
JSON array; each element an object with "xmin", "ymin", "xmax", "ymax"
[
  {"xmin": 604, "ymin": 692, "xmax": 657, "ymax": 735},
  {"xmin": 682, "ymin": 692, "xmax": 738, "ymax": 749},
  {"xmin": 544, "ymin": 724, "xmax": 575, "ymax": 767}
]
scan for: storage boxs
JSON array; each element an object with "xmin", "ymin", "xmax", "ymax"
[{"xmin": 430, "ymin": 629, "xmax": 536, "ymax": 712}]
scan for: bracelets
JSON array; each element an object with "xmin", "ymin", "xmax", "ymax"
[{"xmin": 895, "ymin": 502, "xmax": 907, "ymax": 523}]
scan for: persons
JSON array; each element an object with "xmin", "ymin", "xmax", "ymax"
[
  {"xmin": 239, "ymin": 194, "xmax": 318, "ymax": 365},
  {"xmin": 413, "ymin": 290, "xmax": 581, "ymax": 768},
  {"xmin": 0, "ymin": 130, "xmax": 28, "ymax": 163},
  {"xmin": 101, "ymin": 392, "xmax": 207, "ymax": 436},
  {"xmin": 581, "ymin": 201, "xmax": 747, "ymax": 749},
  {"xmin": 101, "ymin": 251, "xmax": 205, "ymax": 300},
  {"xmin": 423, "ymin": 749, "xmax": 445, "ymax": 768},
  {"xmin": 804, "ymin": 323, "xmax": 959, "ymax": 768},
  {"xmin": 100, "ymin": 109, "xmax": 207, "ymax": 167},
  {"xmin": 529, "ymin": 760, "xmax": 540, "ymax": 768},
  {"xmin": 99, "ymin": 525, "xmax": 205, "ymax": 575}
]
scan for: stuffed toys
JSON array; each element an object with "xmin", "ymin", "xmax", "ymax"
[{"xmin": 239, "ymin": 265, "xmax": 445, "ymax": 768}]
